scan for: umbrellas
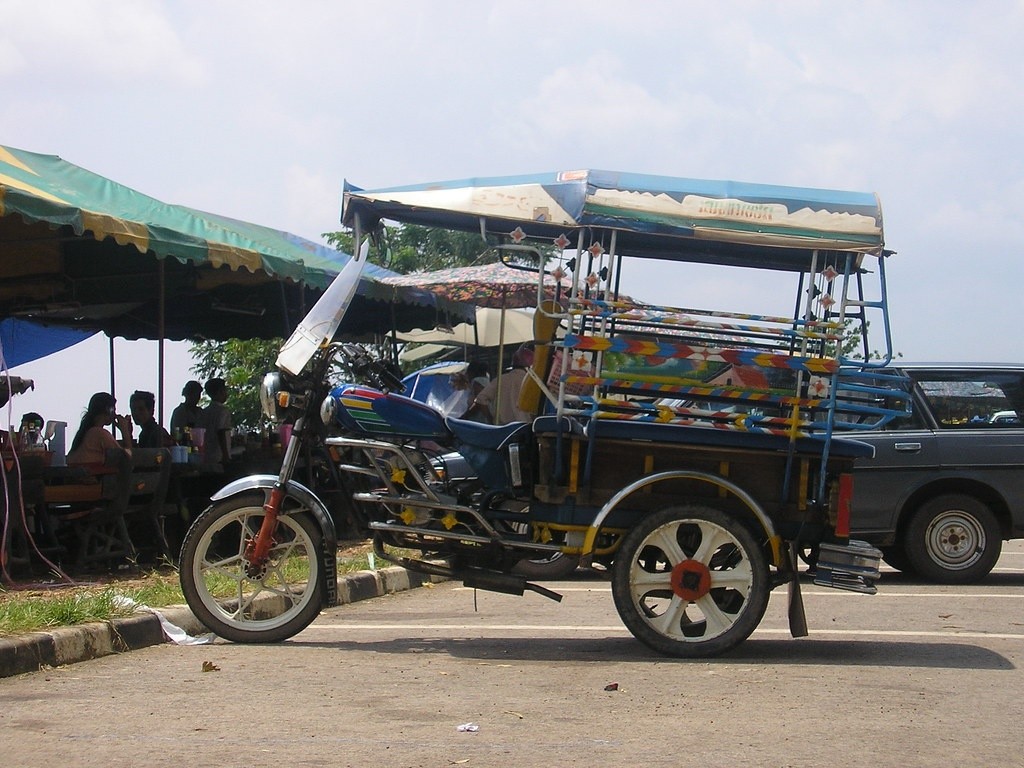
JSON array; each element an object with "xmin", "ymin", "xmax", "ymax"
[{"xmin": 386, "ymin": 256, "xmax": 636, "ymax": 427}]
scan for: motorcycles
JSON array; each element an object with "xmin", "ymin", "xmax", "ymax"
[{"xmin": 178, "ymin": 165, "xmax": 917, "ymax": 662}]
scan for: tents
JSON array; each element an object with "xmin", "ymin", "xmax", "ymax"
[{"xmin": 0, "ymin": 147, "xmax": 474, "ymax": 456}]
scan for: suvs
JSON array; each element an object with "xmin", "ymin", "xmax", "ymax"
[{"xmin": 411, "ymin": 359, "xmax": 1024, "ymax": 588}]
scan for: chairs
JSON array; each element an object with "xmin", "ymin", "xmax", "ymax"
[
  {"xmin": 65, "ymin": 447, "xmax": 173, "ymax": 573},
  {"xmin": 0, "ymin": 456, "xmax": 36, "ymax": 577}
]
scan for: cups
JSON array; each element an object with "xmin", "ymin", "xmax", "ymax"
[{"xmin": 170, "ymin": 446, "xmax": 188, "ymax": 463}]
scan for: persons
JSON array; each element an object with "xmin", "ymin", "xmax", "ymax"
[
  {"xmin": 20, "ymin": 378, "xmax": 232, "ymax": 515},
  {"xmin": 447, "ymin": 345, "xmax": 535, "ymax": 427}
]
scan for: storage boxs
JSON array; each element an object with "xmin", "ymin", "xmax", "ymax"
[{"xmin": 815, "ymin": 540, "xmax": 884, "ymax": 588}]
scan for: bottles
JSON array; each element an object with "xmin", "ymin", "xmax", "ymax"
[
  {"xmin": 6, "ymin": 419, "xmax": 45, "ymax": 452},
  {"xmin": 173, "ymin": 427, "xmax": 198, "ymax": 462}
]
